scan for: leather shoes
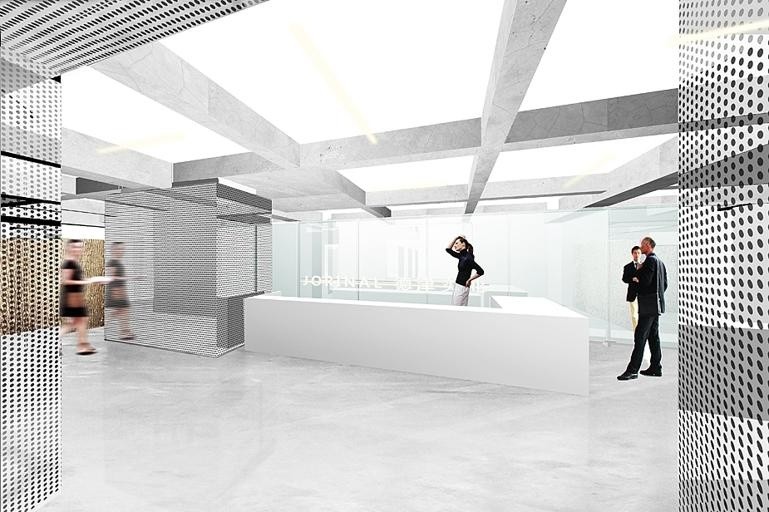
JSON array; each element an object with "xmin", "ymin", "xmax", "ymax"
[
  {"xmin": 640, "ymin": 369, "xmax": 662, "ymax": 376},
  {"xmin": 617, "ymin": 371, "xmax": 637, "ymax": 380}
]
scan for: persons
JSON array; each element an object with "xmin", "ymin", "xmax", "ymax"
[
  {"xmin": 445, "ymin": 235, "xmax": 484, "ymax": 306},
  {"xmin": 616, "ymin": 237, "xmax": 668, "ymax": 381},
  {"xmin": 60, "ymin": 240, "xmax": 95, "ymax": 355},
  {"xmin": 622, "ymin": 245, "xmax": 646, "ymax": 333},
  {"xmin": 104, "ymin": 241, "xmax": 137, "ymax": 339}
]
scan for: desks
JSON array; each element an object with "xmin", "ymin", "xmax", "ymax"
[{"xmin": 243, "ymin": 291, "xmax": 592, "ymax": 398}]
